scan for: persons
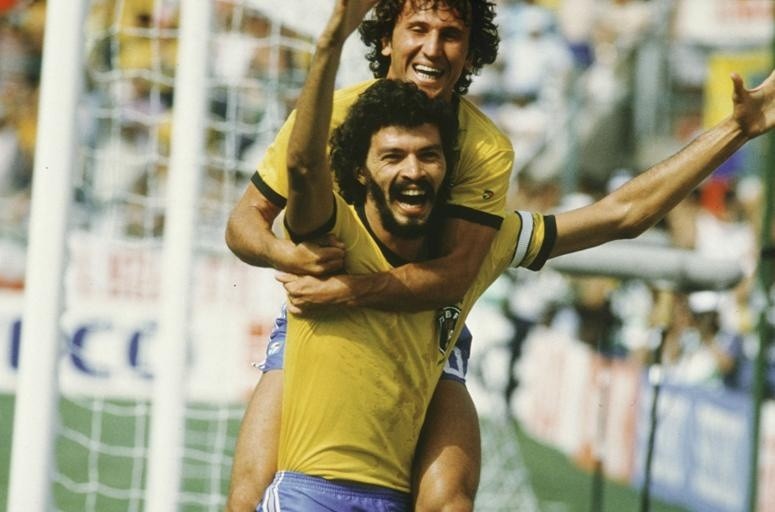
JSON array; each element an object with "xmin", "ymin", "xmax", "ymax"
[
  {"xmin": 225, "ymin": 0, "xmax": 513, "ymax": 512},
  {"xmin": 254, "ymin": 0, "xmax": 775, "ymax": 512}
]
